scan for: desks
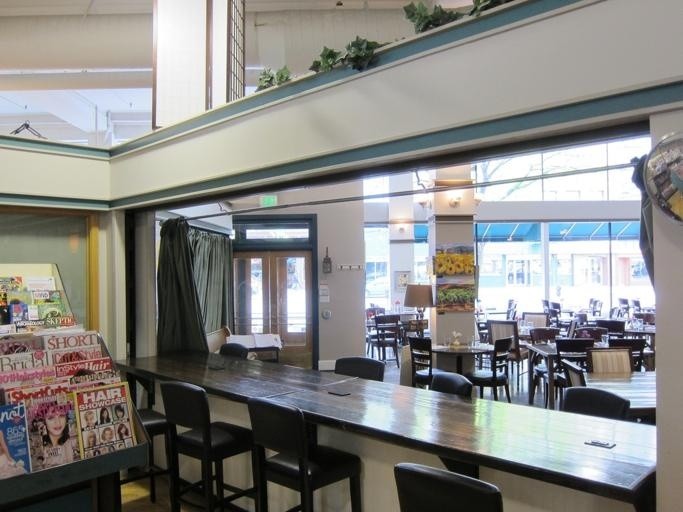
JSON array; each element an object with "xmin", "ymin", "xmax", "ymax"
[
  {"xmin": 584, "ymin": 371, "xmax": 656, "ymax": 414},
  {"xmin": 267, "ymin": 376, "xmax": 658, "ymax": 512},
  {"xmin": 624, "ymin": 324, "xmax": 656, "ymax": 350},
  {"xmin": 112, "ymin": 350, "xmax": 359, "ymax": 512},
  {"xmin": 526, "ymin": 342, "xmax": 656, "ymax": 410},
  {"xmin": 365, "ymin": 322, "xmax": 403, "ymax": 330}
]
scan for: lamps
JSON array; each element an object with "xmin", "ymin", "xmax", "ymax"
[{"xmin": 403, "ymin": 283, "xmax": 434, "ymax": 338}]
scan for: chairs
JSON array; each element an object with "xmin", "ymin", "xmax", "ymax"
[
  {"xmin": 632, "ymin": 298, "xmax": 641, "ymax": 313},
  {"xmin": 334, "ymin": 356, "xmax": 385, "ymax": 383},
  {"xmin": 474, "ymin": 316, "xmax": 489, "ymax": 343},
  {"xmin": 371, "ymin": 314, "xmax": 400, "ymax": 368},
  {"xmin": 248, "ymin": 398, "xmax": 362, "ymax": 512},
  {"xmin": 561, "ymin": 358, "xmax": 587, "ymax": 386},
  {"xmin": 555, "ymin": 338, "xmax": 594, "ymax": 362},
  {"xmin": 592, "ymin": 300, "xmax": 602, "ymax": 317},
  {"xmin": 398, "ymin": 313, "xmax": 417, "ymax": 339},
  {"xmin": 548, "ymin": 309, "xmax": 559, "ymax": 328},
  {"xmin": 585, "ymin": 346, "xmax": 633, "ymax": 375},
  {"xmin": 542, "ymin": 300, "xmax": 549, "ymax": 314},
  {"xmin": 618, "ymin": 298, "xmax": 629, "ymax": 318},
  {"xmin": 393, "ymin": 462, "xmax": 504, "ymax": 512},
  {"xmin": 550, "ymin": 302, "xmax": 561, "ymax": 317},
  {"xmin": 408, "ymin": 336, "xmax": 452, "ymax": 390},
  {"xmin": 574, "ymin": 327, "xmax": 608, "ymax": 340},
  {"xmin": 464, "ymin": 335, "xmax": 514, "ymax": 404},
  {"xmin": 365, "ymin": 308, "xmax": 385, "ymax": 333},
  {"xmin": 522, "ymin": 311, "xmax": 550, "ymax": 329},
  {"xmin": 219, "ymin": 343, "xmax": 249, "ymax": 360},
  {"xmin": 486, "ymin": 320, "xmax": 529, "ymax": 391},
  {"xmin": 558, "ymin": 385, "xmax": 631, "ymax": 422},
  {"xmin": 595, "ymin": 319, "xmax": 625, "ymax": 341},
  {"xmin": 476, "ymin": 309, "xmax": 487, "ymax": 330},
  {"xmin": 573, "ymin": 313, "xmax": 587, "ymax": 326},
  {"xmin": 607, "ymin": 339, "xmax": 646, "ymax": 372},
  {"xmin": 589, "ymin": 298, "xmax": 594, "ymax": 312},
  {"xmin": 429, "ymin": 371, "xmax": 473, "ymax": 397},
  {"xmin": 529, "ymin": 328, "xmax": 560, "ymax": 345},
  {"xmin": 160, "ymin": 380, "xmax": 259, "ymax": 512},
  {"xmin": 506, "ymin": 299, "xmax": 517, "ymax": 321},
  {"xmin": 120, "ymin": 407, "xmax": 181, "ymax": 512},
  {"xmin": 609, "ymin": 306, "xmax": 620, "ymax": 319}
]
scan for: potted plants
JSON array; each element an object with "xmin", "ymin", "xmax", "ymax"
[{"xmin": 436, "ymin": 288, "xmax": 476, "ymax": 315}]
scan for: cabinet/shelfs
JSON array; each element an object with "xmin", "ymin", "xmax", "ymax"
[{"xmin": 0, "ymin": 263, "xmax": 152, "ymax": 512}]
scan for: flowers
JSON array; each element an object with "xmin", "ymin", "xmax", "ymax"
[{"xmin": 434, "ymin": 253, "xmax": 476, "ymax": 276}]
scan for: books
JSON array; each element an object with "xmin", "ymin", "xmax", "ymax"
[{"xmin": 0, "ymin": 272, "xmax": 137, "ymax": 480}]
